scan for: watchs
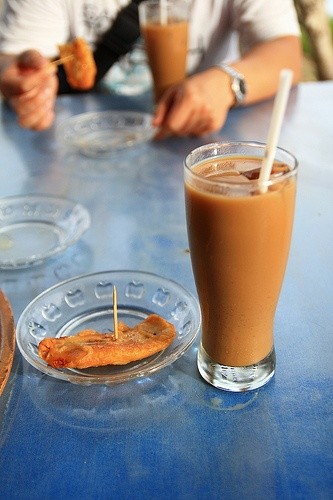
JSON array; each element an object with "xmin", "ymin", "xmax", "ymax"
[{"xmin": 213, "ymin": 63, "xmax": 246, "ymax": 108}]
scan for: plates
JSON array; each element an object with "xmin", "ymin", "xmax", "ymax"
[
  {"xmin": 0, "ymin": 194, "xmax": 89, "ymax": 270},
  {"xmin": 66, "ymin": 110, "xmax": 157, "ymax": 158},
  {"xmin": 16, "ymin": 270, "xmax": 202, "ymax": 384},
  {"xmin": 0, "ymin": 289, "xmax": 16, "ymax": 396}
]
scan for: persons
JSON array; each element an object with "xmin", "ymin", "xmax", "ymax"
[{"xmin": 0, "ymin": 0, "xmax": 304, "ymax": 143}]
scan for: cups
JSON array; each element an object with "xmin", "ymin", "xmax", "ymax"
[
  {"xmin": 138, "ymin": 0, "xmax": 191, "ymax": 118},
  {"xmin": 182, "ymin": 140, "xmax": 298, "ymax": 393}
]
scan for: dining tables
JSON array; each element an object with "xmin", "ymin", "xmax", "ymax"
[{"xmin": 0, "ymin": 78, "xmax": 333, "ymax": 500}]
